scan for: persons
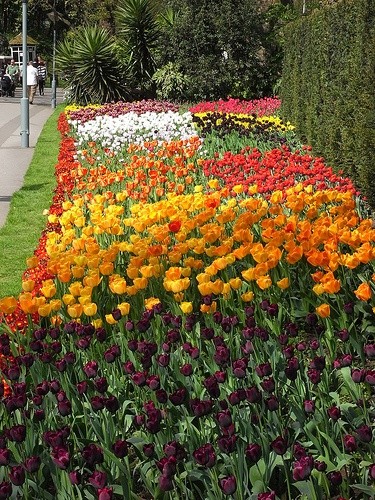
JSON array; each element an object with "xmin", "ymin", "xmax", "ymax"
[
  {"xmin": 36, "ymin": 62, "xmax": 47, "ymax": 95},
  {"xmin": 20, "ymin": 61, "xmax": 39, "ymax": 105},
  {"xmin": 5, "ymin": 59, "xmax": 19, "ymax": 96}
]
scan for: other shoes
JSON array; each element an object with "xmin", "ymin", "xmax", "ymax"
[
  {"xmin": 29, "ymin": 102, "xmax": 33, "ymax": 104},
  {"xmin": 42, "ymin": 92, "xmax": 44, "ymax": 96},
  {"xmin": 39, "ymin": 94, "xmax": 41, "ymax": 96}
]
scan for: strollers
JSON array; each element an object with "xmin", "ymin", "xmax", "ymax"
[{"xmin": 0, "ymin": 75, "xmax": 15, "ymax": 98}]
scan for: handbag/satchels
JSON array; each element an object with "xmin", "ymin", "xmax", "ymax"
[{"xmin": 38, "ymin": 76, "xmax": 43, "ymax": 84}]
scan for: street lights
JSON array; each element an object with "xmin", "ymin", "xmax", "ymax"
[{"xmin": 47, "ymin": 11, "xmax": 65, "ymax": 110}]
